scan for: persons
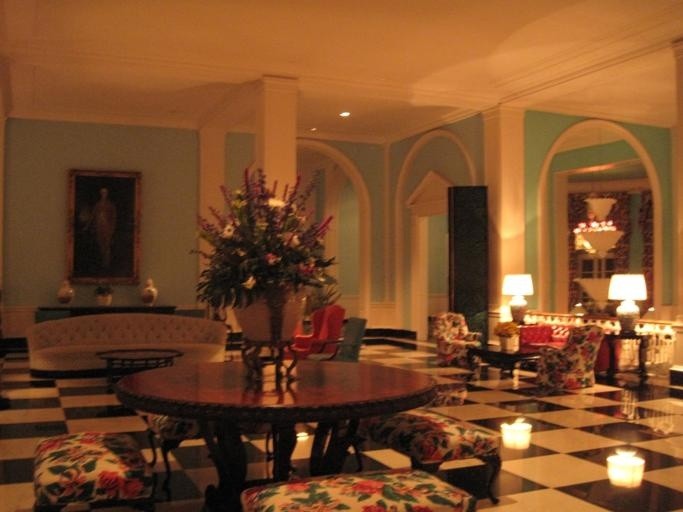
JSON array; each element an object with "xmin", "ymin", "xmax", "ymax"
[{"xmin": 81, "ymin": 187, "xmax": 117, "ymax": 278}]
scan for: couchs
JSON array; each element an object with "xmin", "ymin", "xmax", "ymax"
[
  {"xmin": 519, "ymin": 323, "xmax": 622, "ymax": 375},
  {"xmin": 22, "ymin": 312, "xmax": 229, "ymax": 379}
]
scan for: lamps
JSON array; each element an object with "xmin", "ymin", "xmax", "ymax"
[
  {"xmin": 500, "ymin": 274, "xmax": 534, "ymax": 325},
  {"xmin": 605, "ymin": 448, "xmax": 646, "ymax": 489},
  {"xmin": 500, "ymin": 417, "xmax": 533, "ymax": 451},
  {"xmin": 606, "ymin": 273, "xmax": 648, "ymax": 335}
]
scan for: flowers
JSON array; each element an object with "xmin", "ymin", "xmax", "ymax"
[
  {"xmin": 187, "ymin": 161, "xmax": 336, "ymax": 312},
  {"xmin": 494, "ymin": 321, "xmax": 521, "ymax": 337}
]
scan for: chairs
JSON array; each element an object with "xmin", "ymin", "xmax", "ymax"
[
  {"xmin": 283, "ymin": 304, "xmax": 367, "ymax": 361},
  {"xmin": 428, "ymin": 311, "xmax": 484, "ymax": 370},
  {"xmin": 535, "ymin": 324, "xmax": 605, "ymax": 395}
]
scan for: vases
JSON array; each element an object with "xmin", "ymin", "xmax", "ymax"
[
  {"xmin": 499, "ymin": 334, "xmax": 519, "ymax": 355},
  {"xmin": 230, "ymin": 287, "xmax": 302, "ymax": 344}
]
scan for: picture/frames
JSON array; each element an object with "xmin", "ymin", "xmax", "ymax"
[{"xmin": 67, "ymin": 168, "xmax": 143, "ymax": 286}]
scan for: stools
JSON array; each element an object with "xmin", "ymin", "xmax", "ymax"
[
  {"xmin": 346, "ymin": 406, "xmax": 502, "ymax": 505},
  {"xmin": 240, "ymin": 467, "xmax": 478, "ymax": 512},
  {"xmin": 425, "ymin": 368, "xmax": 476, "ymax": 380},
  {"xmin": 33, "ymin": 430, "xmax": 156, "ymax": 511},
  {"xmin": 430, "ymin": 373, "xmax": 468, "ymax": 407},
  {"xmin": 136, "ymin": 410, "xmax": 279, "ymax": 482}
]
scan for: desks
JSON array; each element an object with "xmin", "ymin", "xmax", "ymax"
[
  {"xmin": 465, "ymin": 340, "xmax": 561, "ymax": 380},
  {"xmin": 112, "ymin": 358, "xmax": 440, "ymax": 512},
  {"xmin": 604, "ymin": 330, "xmax": 652, "ymax": 371},
  {"xmin": 95, "ymin": 347, "xmax": 184, "ymax": 396}
]
